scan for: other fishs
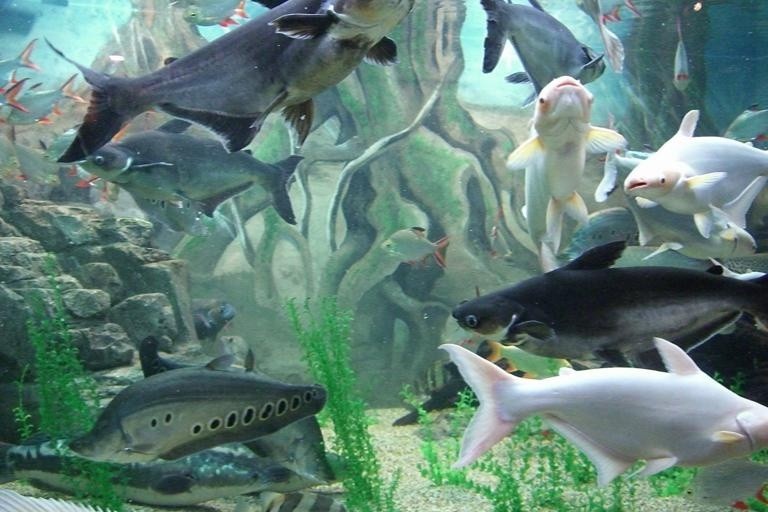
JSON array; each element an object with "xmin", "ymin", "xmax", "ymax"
[
  {"xmin": 437, "ymin": 1, "xmax": 768, "ymax": 511},
  {"xmin": 0, "ymin": 0, "xmax": 417, "ymax": 228},
  {"xmin": 1, "ymin": 361, "xmax": 351, "ymax": 512}
]
